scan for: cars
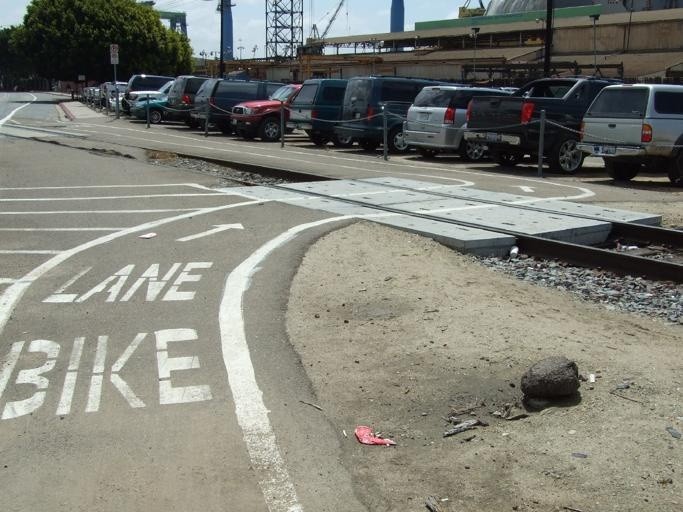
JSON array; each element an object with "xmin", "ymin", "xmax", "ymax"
[
  {"xmin": 498, "ymin": 84, "xmax": 521, "ymax": 94},
  {"xmin": 83, "ymin": 73, "xmax": 186, "ymax": 125}
]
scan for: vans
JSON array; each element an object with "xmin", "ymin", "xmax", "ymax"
[
  {"xmin": 166, "ymin": 75, "xmax": 206, "ymax": 114},
  {"xmin": 398, "ymin": 84, "xmax": 511, "ymax": 164},
  {"xmin": 333, "ymin": 75, "xmax": 441, "ymax": 152}
]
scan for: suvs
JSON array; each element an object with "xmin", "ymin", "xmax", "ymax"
[
  {"xmin": 191, "ymin": 78, "xmax": 289, "ymax": 139},
  {"xmin": 573, "ymin": 81, "xmax": 682, "ymax": 186},
  {"xmin": 227, "ymin": 83, "xmax": 301, "ymax": 141},
  {"xmin": 286, "ymin": 76, "xmax": 357, "ymax": 147}
]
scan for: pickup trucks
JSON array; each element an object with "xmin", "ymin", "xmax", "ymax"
[{"xmin": 461, "ymin": 73, "xmax": 623, "ymax": 173}]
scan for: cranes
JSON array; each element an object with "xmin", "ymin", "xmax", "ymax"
[{"xmin": 305, "ymin": 0, "xmax": 349, "ymax": 45}]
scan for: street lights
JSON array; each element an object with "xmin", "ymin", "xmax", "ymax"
[
  {"xmin": 359, "ymin": 36, "xmax": 386, "ymax": 75},
  {"xmin": 466, "ymin": 25, "xmax": 480, "ymax": 81},
  {"xmin": 535, "ymin": 16, "xmax": 546, "ymax": 65},
  {"xmin": 191, "ymin": 46, "xmax": 257, "ymax": 66},
  {"xmin": 588, "ymin": 13, "xmax": 599, "ymax": 74}
]
